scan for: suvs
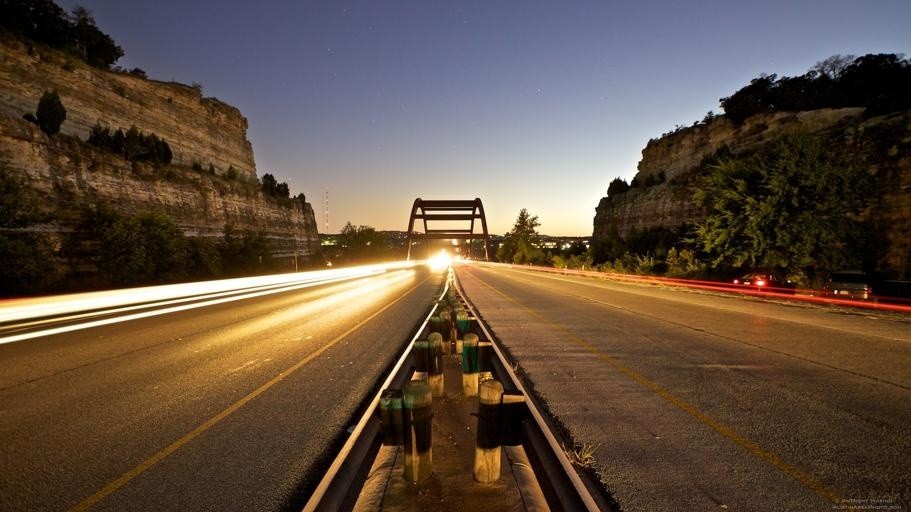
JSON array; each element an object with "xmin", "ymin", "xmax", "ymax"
[{"xmin": 817, "ymin": 268, "xmax": 873, "ymax": 305}]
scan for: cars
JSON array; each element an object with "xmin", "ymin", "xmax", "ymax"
[{"xmin": 732, "ymin": 269, "xmax": 798, "ymax": 301}]
